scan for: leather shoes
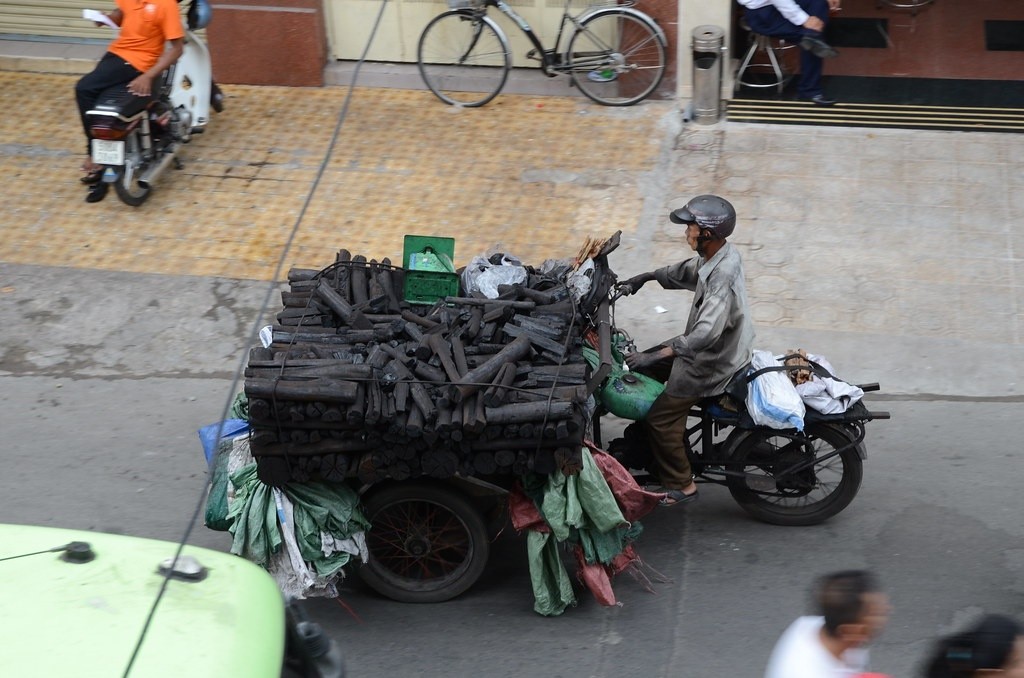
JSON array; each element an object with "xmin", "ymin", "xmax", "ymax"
[
  {"xmin": 799, "ymin": 34, "xmax": 840, "ymax": 59},
  {"xmin": 801, "ymin": 94, "xmax": 836, "ymax": 105}
]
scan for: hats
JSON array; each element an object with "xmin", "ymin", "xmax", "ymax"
[{"xmin": 670, "ymin": 213, "xmax": 693, "ymax": 225}]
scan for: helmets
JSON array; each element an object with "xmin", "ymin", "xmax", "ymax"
[
  {"xmin": 187, "ymin": 0, "xmax": 212, "ymax": 31},
  {"xmin": 675, "ymin": 195, "xmax": 736, "ymax": 239}
]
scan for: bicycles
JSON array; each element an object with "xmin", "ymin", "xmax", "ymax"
[{"xmin": 418, "ymin": 0, "xmax": 664, "ymax": 110}]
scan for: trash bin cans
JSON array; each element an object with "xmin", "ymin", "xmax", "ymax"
[{"xmin": 688, "ymin": 24, "xmax": 728, "ymax": 126}]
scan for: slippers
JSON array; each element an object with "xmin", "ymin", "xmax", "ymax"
[{"xmin": 659, "ymin": 490, "xmax": 699, "ymax": 507}]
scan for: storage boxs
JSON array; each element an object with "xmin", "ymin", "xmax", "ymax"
[{"xmin": 400, "ymin": 235, "xmax": 462, "ymax": 307}]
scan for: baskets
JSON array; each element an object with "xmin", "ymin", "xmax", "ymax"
[{"xmin": 447, "ymin": 0, "xmax": 480, "ymax": 8}]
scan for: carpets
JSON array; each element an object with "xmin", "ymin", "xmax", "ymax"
[
  {"xmin": 827, "ymin": 15, "xmax": 890, "ymax": 49},
  {"xmin": 984, "ymin": 19, "xmax": 1024, "ymax": 52}
]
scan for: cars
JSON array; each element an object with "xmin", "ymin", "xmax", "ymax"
[{"xmin": 1, "ymin": 516, "xmax": 342, "ymax": 678}]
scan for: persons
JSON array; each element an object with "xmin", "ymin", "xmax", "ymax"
[
  {"xmin": 75, "ymin": 1, "xmax": 186, "ymax": 202},
  {"xmin": 738, "ymin": 0, "xmax": 842, "ymax": 103},
  {"xmin": 916, "ymin": 612, "xmax": 1024, "ymax": 678},
  {"xmin": 616, "ymin": 194, "xmax": 755, "ymax": 507},
  {"xmin": 763, "ymin": 570, "xmax": 895, "ymax": 678}
]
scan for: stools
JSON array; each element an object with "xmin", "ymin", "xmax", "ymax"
[{"xmin": 735, "ymin": 19, "xmax": 797, "ymax": 93}]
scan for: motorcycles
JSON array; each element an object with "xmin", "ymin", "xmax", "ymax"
[
  {"xmin": 76, "ymin": 0, "xmax": 227, "ymax": 207},
  {"xmin": 264, "ymin": 232, "xmax": 866, "ymax": 601}
]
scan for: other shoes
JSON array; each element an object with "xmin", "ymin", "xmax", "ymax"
[
  {"xmin": 87, "ymin": 181, "xmax": 108, "ymax": 202},
  {"xmin": 81, "ymin": 170, "xmax": 103, "ymax": 184}
]
